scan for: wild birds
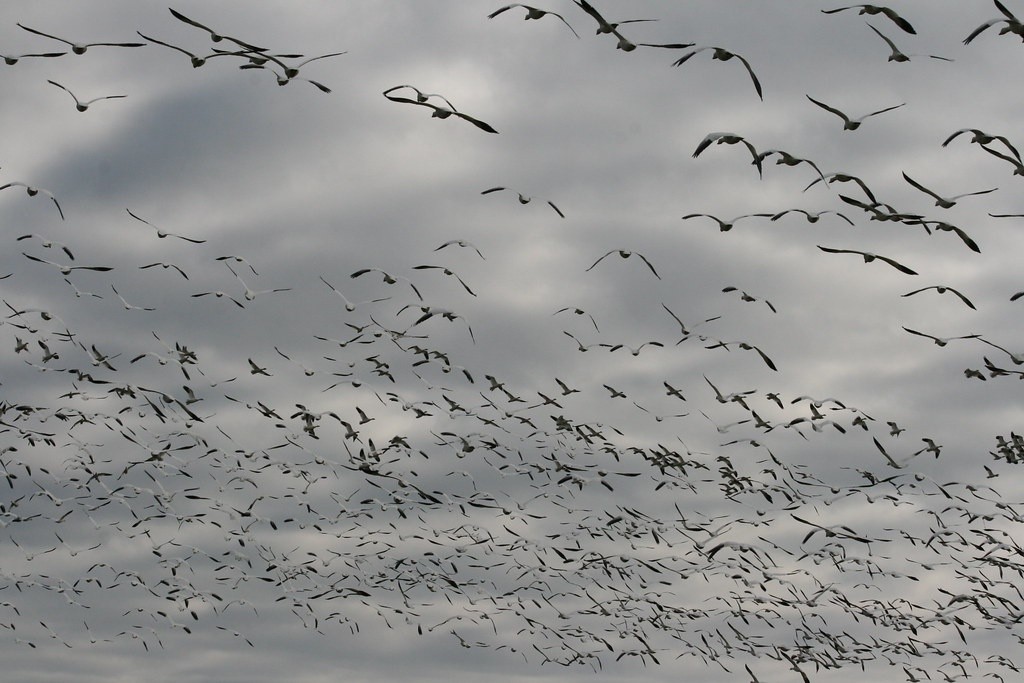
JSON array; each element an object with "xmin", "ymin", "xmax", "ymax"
[{"xmin": 0, "ymin": 0, "xmax": 1024, "ymax": 682}]
